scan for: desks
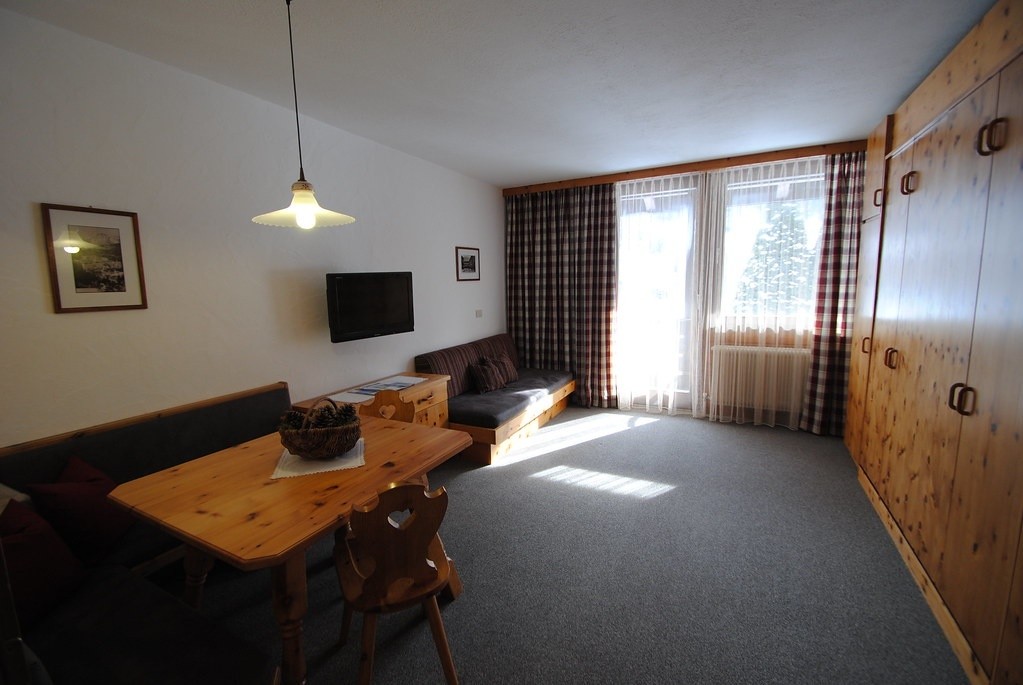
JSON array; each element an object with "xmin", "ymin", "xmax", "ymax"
[{"xmin": 107, "ymin": 415, "xmax": 473, "ymax": 685}]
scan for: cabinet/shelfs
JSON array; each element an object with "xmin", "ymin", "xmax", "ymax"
[
  {"xmin": 845, "ymin": 0, "xmax": 1023, "ymax": 685},
  {"xmin": 292, "ymin": 372, "xmax": 451, "ymax": 429}
]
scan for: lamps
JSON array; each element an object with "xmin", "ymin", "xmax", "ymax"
[{"xmin": 251, "ymin": 1, "xmax": 355, "ymax": 229}]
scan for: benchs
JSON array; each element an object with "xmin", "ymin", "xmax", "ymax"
[
  {"xmin": 0, "ymin": 382, "xmax": 293, "ymax": 641},
  {"xmin": 415, "ymin": 334, "xmax": 576, "ymax": 465}
]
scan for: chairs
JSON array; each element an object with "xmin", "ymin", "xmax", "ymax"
[{"xmin": 332, "ymin": 479, "xmax": 459, "ymax": 685}]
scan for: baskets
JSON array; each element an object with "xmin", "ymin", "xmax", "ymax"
[{"xmin": 280, "ymin": 397, "xmax": 361, "ymax": 460}]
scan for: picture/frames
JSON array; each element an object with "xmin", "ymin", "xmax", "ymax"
[
  {"xmin": 41, "ymin": 203, "xmax": 148, "ymax": 314},
  {"xmin": 455, "ymin": 247, "xmax": 480, "ymax": 281}
]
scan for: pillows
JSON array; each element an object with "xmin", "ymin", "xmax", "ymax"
[
  {"xmin": 0, "ymin": 499, "xmax": 84, "ymax": 631},
  {"xmin": 492, "ymin": 353, "xmax": 518, "ymax": 384},
  {"xmin": 468, "ymin": 356, "xmax": 506, "ymax": 394},
  {"xmin": 26, "ymin": 455, "xmax": 135, "ymax": 563}
]
